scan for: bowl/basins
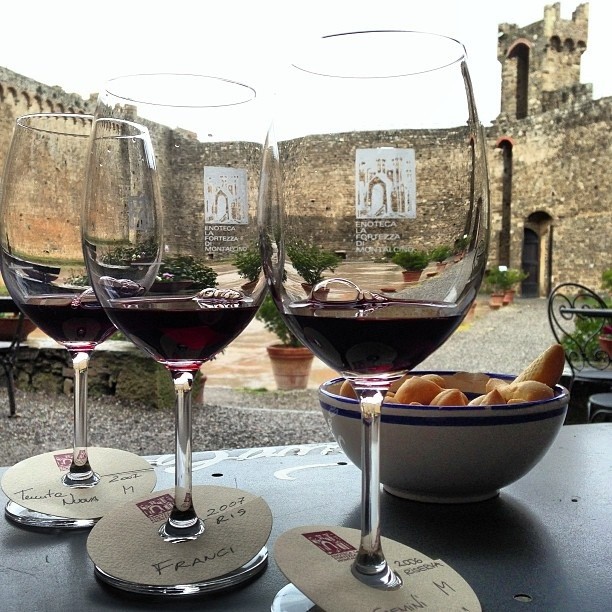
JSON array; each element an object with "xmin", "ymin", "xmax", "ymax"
[{"xmin": 318, "ymin": 369, "xmax": 572, "ymax": 505}]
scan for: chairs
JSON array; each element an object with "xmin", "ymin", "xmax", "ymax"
[
  {"xmin": 547, "ymin": 279, "xmax": 611, "ymax": 425},
  {"xmin": 583, "ymin": 390, "xmax": 611, "ymax": 425}
]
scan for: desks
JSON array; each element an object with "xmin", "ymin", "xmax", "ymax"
[
  {"xmin": 560, "ymin": 305, "xmax": 611, "ymax": 322},
  {"xmin": 0, "ymin": 418, "xmax": 612, "ymax": 611}
]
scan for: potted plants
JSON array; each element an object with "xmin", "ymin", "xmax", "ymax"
[
  {"xmin": 501, "ymin": 269, "xmax": 528, "ymax": 303},
  {"xmin": 586, "ymin": 298, "xmax": 612, "ymax": 360},
  {"xmin": 453, "ymin": 235, "xmax": 473, "ymax": 262},
  {"xmin": 1, "ymin": 285, "xmax": 38, "ymax": 343},
  {"xmin": 592, "ymin": 266, "xmax": 612, "ymax": 334},
  {"xmin": 235, "ymin": 238, "xmax": 343, "ymax": 391},
  {"xmin": 486, "ymin": 270, "xmax": 509, "ymax": 307},
  {"xmin": 386, "ymin": 247, "xmax": 427, "ymax": 284},
  {"xmin": 429, "ymin": 244, "xmax": 453, "ymax": 274}
]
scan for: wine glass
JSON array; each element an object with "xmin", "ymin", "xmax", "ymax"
[
  {"xmin": 256, "ymin": 29, "xmax": 491, "ymax": 612},
  {"xmin": 81, "ymin": 73, "xmax": 268, "ymax": 599},
  {"xmin": 0, "ymin": 111, "xmax": 164, "ymax": 533}
]
